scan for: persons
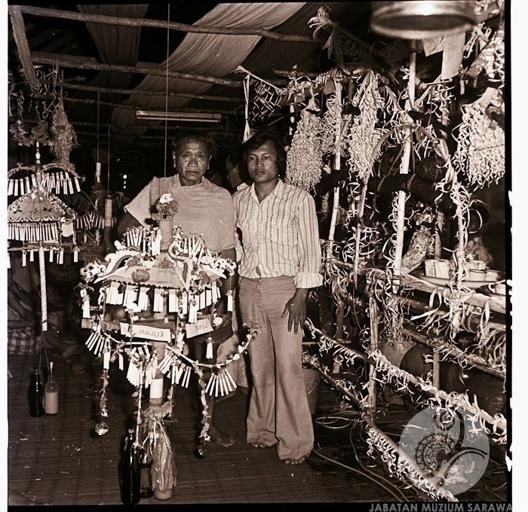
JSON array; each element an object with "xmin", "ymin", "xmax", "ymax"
[
  {"xmin": 210, "ymin": 149, "xmax": 253, "ymax": 403},
  {"xmin": 231, "ymin": 132, "xmax": 325, "ymax": 467},
  {"xmin": 115, "ymin": 126, "xmax": 249, "ymax": 450},
  {"xmin": 7, "ymin": 180, "xmax": 126, "ymax": 369}
]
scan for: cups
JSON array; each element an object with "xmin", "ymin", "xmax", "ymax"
[{"xmin": 488, "ymin": 279, "xmax": 506, "ymax": 296}]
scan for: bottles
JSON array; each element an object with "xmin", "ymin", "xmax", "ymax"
[
  {"xmin": 118, "ymin": 425, "xmax": 154, "ymax": 505},
  {"xmin": 43, "ymin": 361, "xmax": 59, "ymax": 416},
  {"xmin": 27, "ymin": 369, "xmax": 44, "ymax": 418}
]
